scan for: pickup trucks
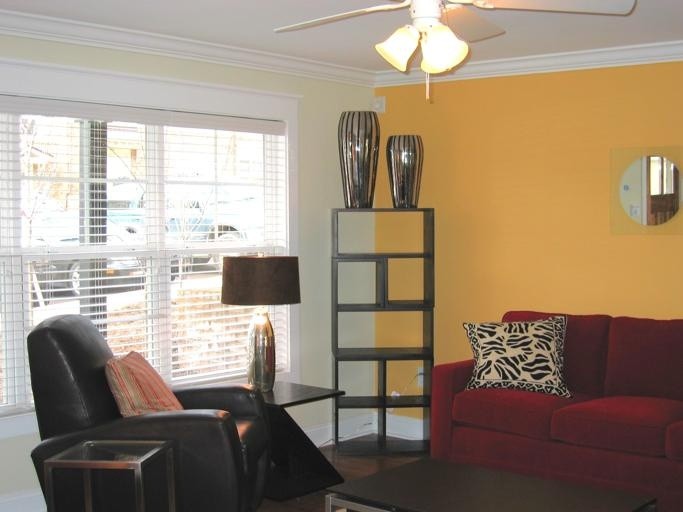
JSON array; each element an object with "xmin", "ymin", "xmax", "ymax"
[{"xmin": 106, "ymin": 187, "xmax": 289, "ymax": 272}]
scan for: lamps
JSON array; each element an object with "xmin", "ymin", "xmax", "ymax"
[
  {"xmin": 376, "ymin": 1, "xmax": 471, "ymax": 74},
  {"xmin": 222, "ymin": 256, "xmax": 301, "ymax": 393}
]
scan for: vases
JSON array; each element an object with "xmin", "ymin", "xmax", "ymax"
[{"xmin": 338, "ymin": 111, "xmax": 425, "ymax": 209}]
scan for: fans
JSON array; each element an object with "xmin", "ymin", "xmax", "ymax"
[{"xmin": 274, "ymin": 0, "xmax": 635, "ymax": 42}]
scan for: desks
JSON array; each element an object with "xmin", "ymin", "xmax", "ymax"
[
  {"xmin": 240, "ymin": 381, "xmax": 346, "ymax": 501},
  {"xmin": 45, "ymin": 441, "xmax": 178, "ymax": 511}
]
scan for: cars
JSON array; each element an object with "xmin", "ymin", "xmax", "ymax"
[{"xmin": 26, "ymin": 236, "xmax": 145, "ymax": 300}]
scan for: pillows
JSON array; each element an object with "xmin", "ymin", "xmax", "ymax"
[
  {"xmin": 461, "ymin": 315, "xmax": 569, "ymax": 397},
  {"xmin": 105, "ymin": 351, "xmax": 185, "ymax": 421}
]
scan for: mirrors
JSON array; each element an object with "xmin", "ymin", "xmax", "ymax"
[{"xmin": 610, "ymin": 146, "xmax": 683, "ymax": 234}]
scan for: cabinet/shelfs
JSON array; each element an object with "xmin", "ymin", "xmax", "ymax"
[{"xmin": 331, "ymin": 208, "xmax": 435, "ymax": 458}]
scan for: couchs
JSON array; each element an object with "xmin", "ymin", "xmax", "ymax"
[{"xmin": 431, "ymin": 310, "xmax": 682, "ymax": 511}]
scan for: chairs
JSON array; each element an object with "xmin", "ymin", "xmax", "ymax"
[{"xmin": 28, "ymin": 314, "xmax": 273, "ymax": 512}]
